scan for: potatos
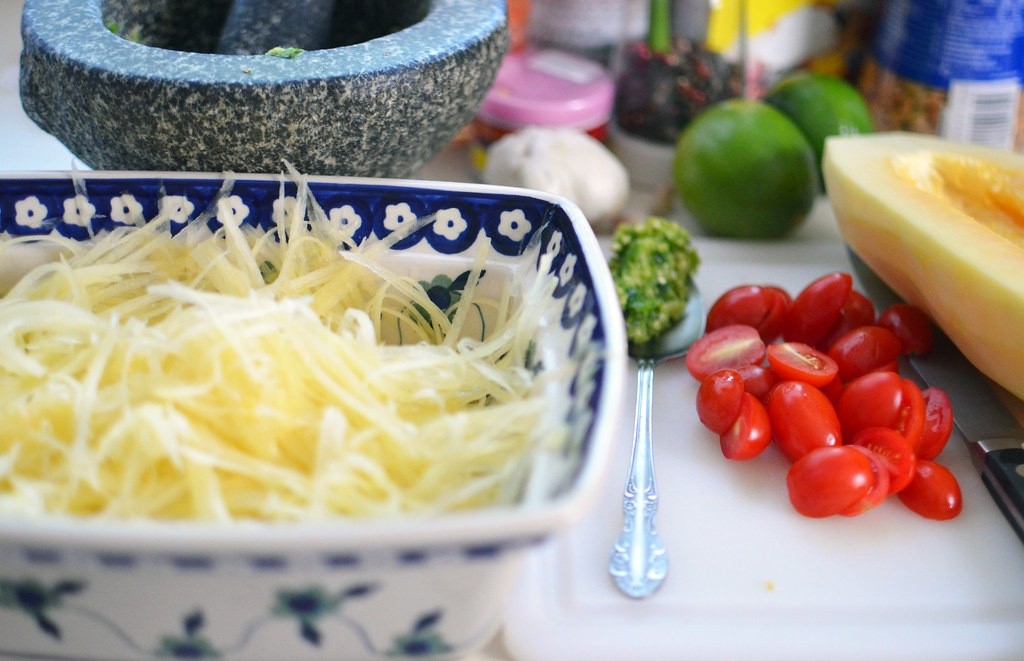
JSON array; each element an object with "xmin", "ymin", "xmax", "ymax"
[{"xmin": 0, "ymin": 156, "xmax": 565, "ymax": 526}]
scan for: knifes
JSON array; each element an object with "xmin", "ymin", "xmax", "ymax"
[{"xmin": 846, "ymin": 245, "xmax": 1023, "ymax": 549}]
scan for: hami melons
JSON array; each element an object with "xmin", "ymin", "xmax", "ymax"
[{"xmin": 825, "ymin": 132, "xmax": 1024, "ymax": 401}]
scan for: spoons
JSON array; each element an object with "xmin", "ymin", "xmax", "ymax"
[{"xmin": 607, "ymin": 273, "xmax": 707, "ymax": 600}]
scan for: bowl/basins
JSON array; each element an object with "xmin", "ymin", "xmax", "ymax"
[{"xmin": 0, "ymin": 169, "xmax": 626, "ymax": 661}]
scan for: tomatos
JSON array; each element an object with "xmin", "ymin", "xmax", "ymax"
[{"xmin": 686, "ymin": 272, "xmax": 963, "ymax": 519}]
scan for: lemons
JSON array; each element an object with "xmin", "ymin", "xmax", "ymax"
[{"xmin": 673, "ymin": 72, "xmax": 874, "ymax": 240}]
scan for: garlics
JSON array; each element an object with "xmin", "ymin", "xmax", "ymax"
[{"xmin": 480, "ymin": 125, "xmax": 629, "ymax": 231}]
